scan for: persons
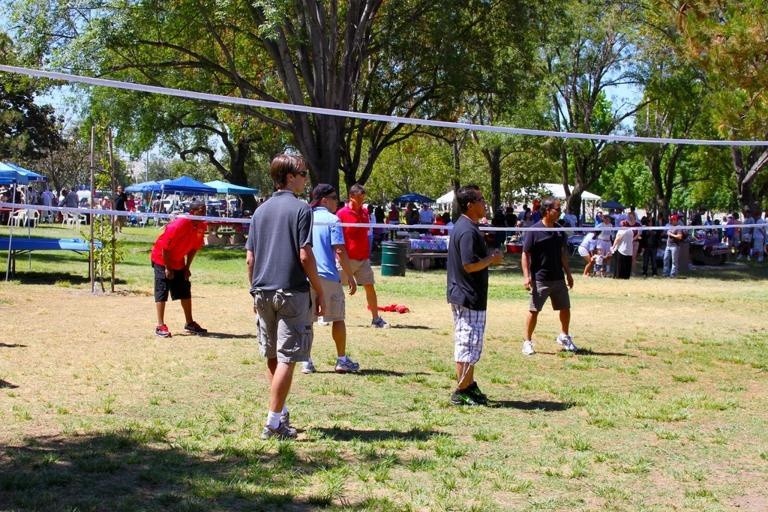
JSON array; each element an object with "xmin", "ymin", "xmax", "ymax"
[
  {"xmin": 302, "ymin": 184, "xmax": 359, "ymax": 375},
  {"xmin": 319, "ymin": 184, "xmax": 392, "ymax": 328},
  {"xmin": 245, "ymin": 154, "xmax": 325, "ymax": 440},
  {"xmin": 364, "ymin": 204, "xmax": 455, "ymax": 251},
  {"xmin": 490, "ymin": 204, "xmax": 542, "ymax": 251},
  {"xmin": 0, "ymin": 183, "xmax": 89, "ymax": 227},
  {"xmin": 557, "ymin": 206, "xmax": 768, "ymax": 278},
  {"xmin": 447, "ymin": 184, "xmax": 503, "ymax": 408},
  {"xmin": 125, "ymin": 184, "xmax": 258, "ymax": 231},
  {"xmin": 521, "ymin": 194, "xmax": 577, "ymax": 352},
  {"xmin": 95, "ymin": 186, "xmax": 128, "ymax": 232},
  {"xmin": 150, "ymin": 199, "xmax": 207, "ymax": 336}
]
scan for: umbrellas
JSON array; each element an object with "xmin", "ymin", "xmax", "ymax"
[
  {"xmin": 596, "ymin": 201, "xmax": 624, "ymax": 209},
  {"xmin": 392, "ymin": 193, "xmax": 436, "ymax": 202}
]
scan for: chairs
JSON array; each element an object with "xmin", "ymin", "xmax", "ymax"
[
  {"xmin": 67, "ymin": 211, "xmax": 87, "ymax": 233},
  {"xmin": 7, "ymin": 209, "xmax": 29, "ymax": 228},
  {"xmin": 22, "ymin": 209, "xmax": 40, "ymax": 228},
  {"xmin": 61, "ymin": 211, "xmax": 73, "ymax": 228}
]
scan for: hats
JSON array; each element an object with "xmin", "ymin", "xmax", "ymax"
[{"xmin": 310, "ymin": 184, "xmax": 332, "ymax": 207}]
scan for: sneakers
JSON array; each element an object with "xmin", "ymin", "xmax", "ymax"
[
  {"xmin": 301, "ymin": 362, "xmax": 316, "ymax": 373},
  {"xmin": 557, "ymin": 334, "xmax": 576, "ymax": 351},
  {"xmin": 184, "ymin": 321, "xmax": 207, "ymax": 333},
  {"xmin": 335, "ymin": 356, "xmax": 359, "ymax": 372},
  {"xmin": 261, "ymin": 411, "xmax": 296, "ymax": 440},
  {"xmin": 156, "ymin": 324, "xmax": 170, "ymax": 338},
  {"xmin": 371, "ymin": 318, "xmax": 390, "ymax": 328},
  {"xmin": 522, "ymin": 341, "xmax": 534, "ymax": 355},
  {"xmin": 318, "ymin": 316, "xmax": 328, "ymax": 326},
  {"xmin": 451, "ymin": 382, "xmax": 486, "ymax": 405}
]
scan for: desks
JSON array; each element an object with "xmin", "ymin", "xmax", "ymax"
[{"xmin": 0, "ymin": 236, "xmax": 102, "ymax": 282}]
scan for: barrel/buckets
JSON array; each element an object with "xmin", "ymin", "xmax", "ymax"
[{"xmin": 380, "ymin": 240, "xmax": 409, "ymax": 277}]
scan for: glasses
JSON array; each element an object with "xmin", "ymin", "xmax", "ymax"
[{"xmin": 298, "ymin": 171, "xmax": 307, "ymax": 176}]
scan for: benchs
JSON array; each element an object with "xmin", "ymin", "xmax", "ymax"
[{"xmin": 409, "ymin": 240, "xmax": 450, "ymax": 271}]
